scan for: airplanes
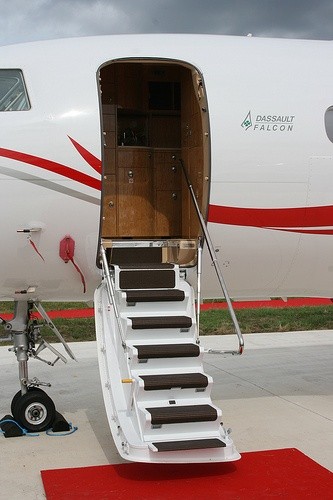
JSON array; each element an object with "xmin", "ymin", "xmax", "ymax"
[{"xmin": 0, "ymin": 31, "xmax": 332, "ymax": 465}]
